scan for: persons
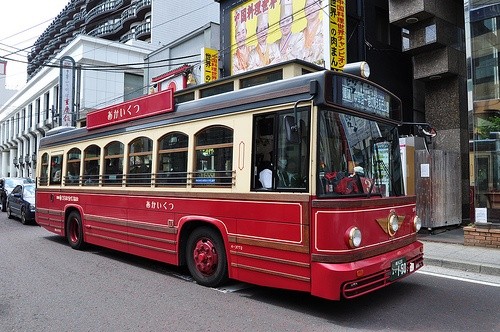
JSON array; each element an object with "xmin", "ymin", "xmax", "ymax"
[
  {"xmin": 271, "ymin": 6, "xmax": 309, "ymax": 63},
  {"xmin": 45, "ymin": 157, "xmax": 122, "ymax": 186},
  {"xmin": 260, "ymin": 147, "xmax": 294, "ymax": 189},
  {"xmin": 231, "ymin": 21, "xmax": 255, "ymax": 73},
  {"xmin": 300, "ymin": 0, "xmax": 330, "ymax": 69},
  {"xmin": 248, "ymin": 12, "xmax": 282, "ymax": 69}
]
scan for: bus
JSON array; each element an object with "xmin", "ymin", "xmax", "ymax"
[{"xmin": 34, "ymin": 59, "xmax": 437, "ymax": 301}]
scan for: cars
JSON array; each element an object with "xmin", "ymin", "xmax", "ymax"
[
  {"xmin": 0, "ymin": 177, "xmax": 32, "ymax": 211},
  {"xmin": 6, "ymin": 183, "xmax": 35, "ymax": 225}
]
